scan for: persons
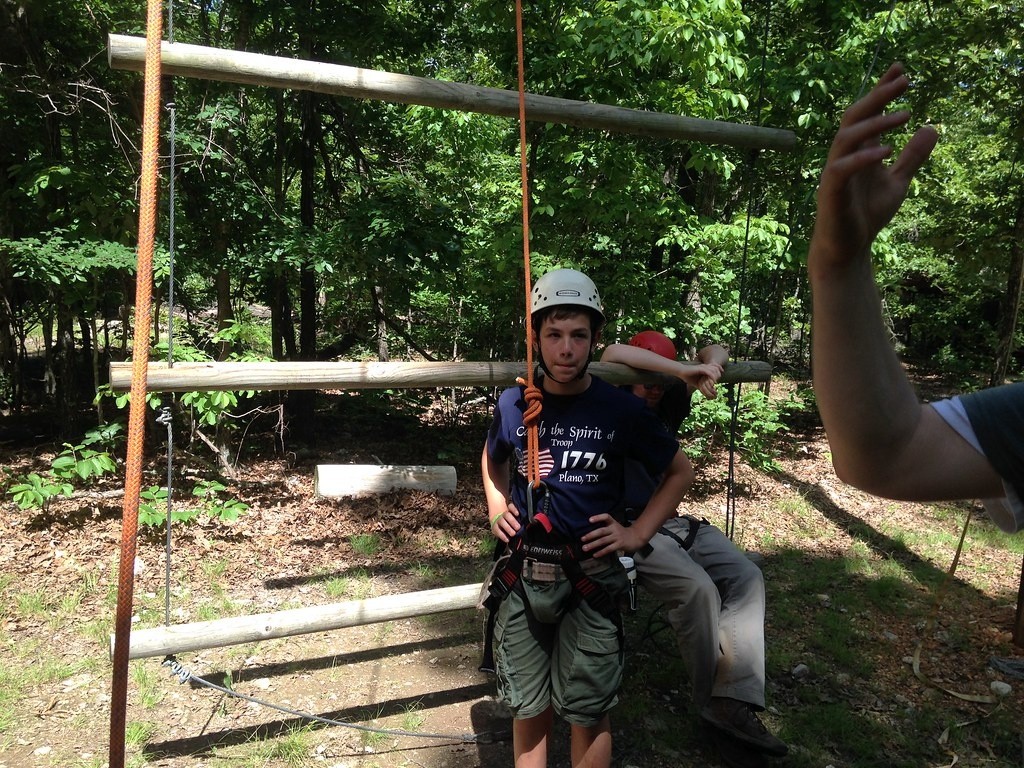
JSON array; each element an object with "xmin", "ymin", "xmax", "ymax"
[
  {"xmin": 600, "ymin": 331, "xmax": 788, "ymax": 767},
  {"xmin": 482, "ymin": 269, "xmax": 694, "ymax": 768},
  {"xmin": 807, "ymin": 64, "xmax": 1024, "ymax": 532}
]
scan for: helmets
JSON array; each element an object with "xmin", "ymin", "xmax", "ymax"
[
  {"xmin": 628, "ymin": 331, "xmax": 676, "ymax": 361},
  {"xmin": 523, "ymin": 268, "xmax": 606, "ymax": 330}
]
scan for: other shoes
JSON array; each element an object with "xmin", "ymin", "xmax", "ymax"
[{"xmin": 693, "ymin": 696, "xmax": 789, "ymax": 768}]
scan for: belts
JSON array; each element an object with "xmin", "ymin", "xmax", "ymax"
[{"xmin": 502, "ymin": 546, "xmax": 616, "ymax": 582}]
